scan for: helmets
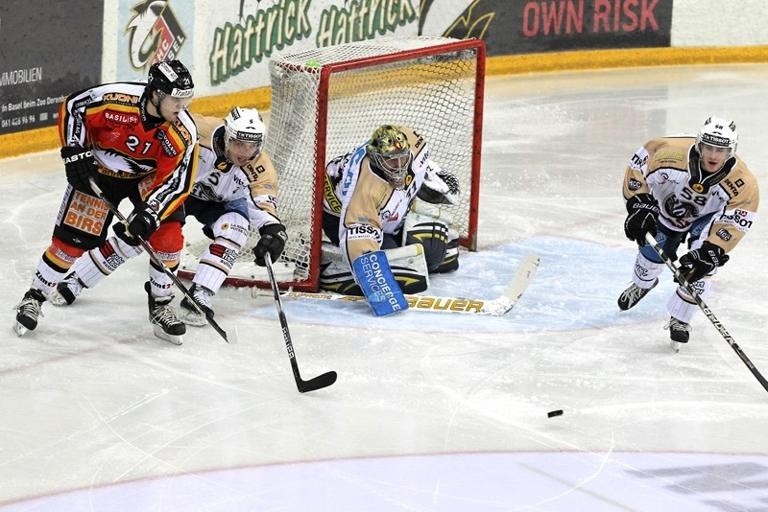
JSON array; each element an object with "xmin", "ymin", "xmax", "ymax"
[
  {"xmin": 141, "ymin": 58, "xmax": 196, "ymax": 107},
  {"xmin": 362, "ymin": 125, "xmax": 412, "ymax": 187},
  {"xmin": 221, "ymin": 105, "xmax": 265, "ymax": 163},
  {"xmin": 695, "ymin": 117, "xmax": 740, "ymax": 165}
]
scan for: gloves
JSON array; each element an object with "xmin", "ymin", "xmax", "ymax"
[
  {"xmin": 252, "ymin": 222, "xmax": 288, "ymax": 267},
  {"xmin": 624, "ymin": 193, "xmax": 661, "ymax": 247},
  {"xmin": 58, "ymin": 143, "xmax": 104, "ymax": 193},
  {"xmin": 111, "ymin": 203, "xmax": 162, "ymax": 247},
  {"xmin": 672, "ymin": 241, "xmax": 730, "ymax": 283}
]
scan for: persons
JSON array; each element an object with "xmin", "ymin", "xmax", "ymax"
[
  {"xmin": 49, "ymin": 106, "xmax": 288, "ymax": 328},
  {"xmin": 618, "ymin": 116, "xmax": 759, "ymax": 351},
  {"xmin": 15, "ymin": 60, "xmax": 199, "ymax": 345},
  {"xmin": 323, "ymin": 124, "xmax": 461, "ymax": 317}
]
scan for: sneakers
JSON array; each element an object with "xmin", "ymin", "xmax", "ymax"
[
  {"xmin": 12, "ymin": 287, "xmax": 46, "ymax": 331},
  {"xmin": 52, "ymin": 272, "xmax": 85, "ymax": 308},
  {"xmin": 661, "ymin": 313, "xmax": 692, "ymax": 345},
  {"xmin": 616, "ymin": 276, "xmax": 661, "ymax": 313},
  {"xmin": 143, "ymin": 280, "xmax": 187, "ymax": 336},
  {"xmin": 179, "ymin": 283, "xmax": 215, "ymax": 319}
]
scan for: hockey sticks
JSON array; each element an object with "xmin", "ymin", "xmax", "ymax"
[
  {"xmin": 264, "ymin": 248, "xmax": 337, "ymax": 394},
  {"xmin": 277, "ymin": 251, "xmax": 540, "ymax": 306}
]
